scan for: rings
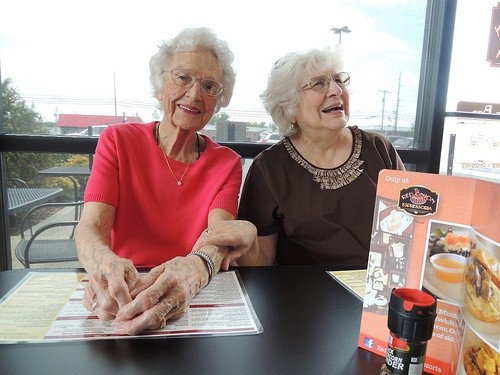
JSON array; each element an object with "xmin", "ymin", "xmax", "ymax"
[
  {"xmin": 160, "ymin": 318, "xmax": 167, "ymax": 329},
  {"xmin": 92, "ymin": 301, "xmax": 98, "ymax": 311},
  {"xmin": 204, "ymin": 229, "xmax": 213, "ymax": 235}
]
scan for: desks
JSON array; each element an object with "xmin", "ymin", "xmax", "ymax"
[
  {"xmin": 0, "ymin": 265, "xmax": 386, "ymax": 375},
  {"xmin": 38, "ymin": 166, "xmax": 91, "ymax": 239},
  {"xmin": 7, "ymin": 187, "xmax": 64, "ymax": 215}
]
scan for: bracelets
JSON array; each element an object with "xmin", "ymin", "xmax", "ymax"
[{"xmin": 187, "ymin": 250, "xmax": 216, "ymax": 288}]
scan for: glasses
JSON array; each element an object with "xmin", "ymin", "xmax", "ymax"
[
  {"xmin": 163, "ymin": 69, "xmax": 226, "ymax": 96},
  {"xmin": 300, "ymin": 72, "xmax": 350, "ymax": 91}
]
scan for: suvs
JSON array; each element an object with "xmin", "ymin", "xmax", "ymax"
[{"xmin": 258, "ymin": 132, "xmax": 282, "ymax": 144}]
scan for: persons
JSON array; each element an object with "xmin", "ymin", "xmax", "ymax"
[
  {"xmin": 74, "ymin": 26, "xmax": 243, "ymax": 334},
  {"xmin": 191, "ymin": 50, "xmax": 407, "ymax": 271}
]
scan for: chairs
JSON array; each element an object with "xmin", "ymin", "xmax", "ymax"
[{"xmin": 15, "ymin": 201, "xmax": 83, "ymax": 268}]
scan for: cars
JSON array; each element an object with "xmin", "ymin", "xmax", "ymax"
[
  {"xmin": 392, "ymin": 137, "xmax": 414, "ymax": 149},
  {"xmin": 65, "ymin": 125, "xmax": 107, "ymax": 137}
]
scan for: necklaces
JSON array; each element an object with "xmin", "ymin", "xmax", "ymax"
[{"xmin": 155, "ymin": 126, "xmax": 201, "ymax": 186}]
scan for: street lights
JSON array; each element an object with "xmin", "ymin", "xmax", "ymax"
[{"xmin": 330, "ymin": 26, "xmax": 352, "ymax": 62}]
